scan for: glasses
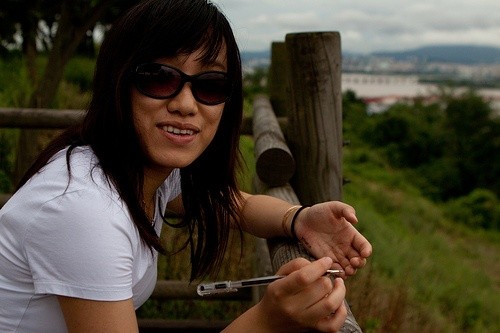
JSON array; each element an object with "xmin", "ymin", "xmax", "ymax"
[{"xmin": 128, "ymin": 60, "xmax": 239, "ymax": 106}]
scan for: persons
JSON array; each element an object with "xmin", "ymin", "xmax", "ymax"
[{"xmin": 0, "ymin": 0, "xmax": 373, "ymax": 333}]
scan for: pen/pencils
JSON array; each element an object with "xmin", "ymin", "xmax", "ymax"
[{"xmin": 197, "ymin": 267, "xmax": 345, "ymax": 298}]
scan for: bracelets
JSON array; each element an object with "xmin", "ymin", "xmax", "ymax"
[{"xmin": 281, "ymin": 204, "xmax": 313, "ymax": 243}]
